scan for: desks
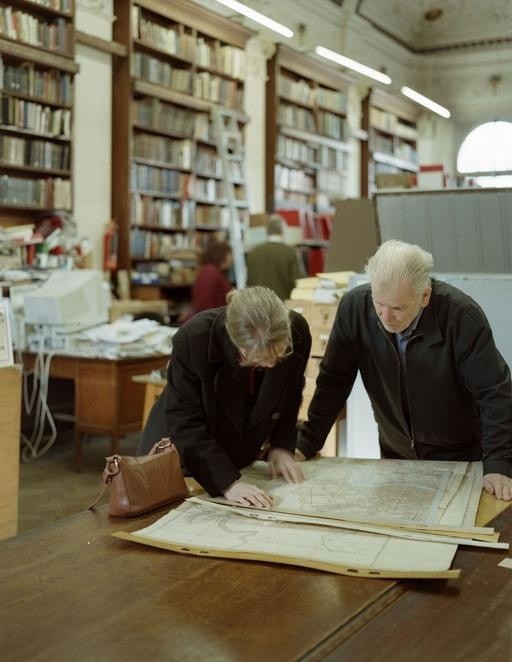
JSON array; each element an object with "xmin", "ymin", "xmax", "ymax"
[{"xmin": 0, "ymin": 500, "xmax": 512, "ymax": 662}]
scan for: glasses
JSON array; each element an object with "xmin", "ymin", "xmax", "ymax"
[{"xmin": 239, "ymin": 335, "xmax": 294, "ymax": 368}]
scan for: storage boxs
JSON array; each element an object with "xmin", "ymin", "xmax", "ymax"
[
  {"xmin": 296, "ymin": 358, "xmax": 338, "ymax": 460},
  {"xmin": 283, "ymin": 298, "xmax": 338, "ymax": 358}
]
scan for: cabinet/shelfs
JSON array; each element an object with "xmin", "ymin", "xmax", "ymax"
[
  {"xmin": 0, "ymin": 364, "xmax": 26, "ymax": 545},
  {"xmin": 109, "ymin": 1, "xmax": 276, "ymax": 302},
  {"xmin": 359, "ymin": 86, "xmax": 421, "ymax": 198},
  {"xmin": 0, "ymin": 0, "xmax": 79, "ymax": 228},
  {"xmin": 13, "ymin": 350, "xmax": 172, "ymax": 456},
  {"xmin": 265, "ymin": 42, "xmax": 360, "ymax": 215}
]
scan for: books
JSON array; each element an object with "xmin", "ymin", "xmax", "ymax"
[
  {"xmin": 274, "ymin": 74, "xmax": 349, "ymax": 242},
  {"xmin": 0, "ymin": 0, "xmax": 76, "ymax": 271},
  {"xmin": 129, "ymin": 6, "xmax": 248, "ymax": 262},
  {"xmin": 369, "ymin": 106, "xmax": 419, "ymax": 189}
]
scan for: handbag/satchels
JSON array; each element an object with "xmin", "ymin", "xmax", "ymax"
[{"xmin": 105, "ymin": 439, "xmax": 190, "ymax": 518}]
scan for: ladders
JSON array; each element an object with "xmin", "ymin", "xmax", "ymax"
[{"xmin": 211, "ymin": 104, "xmax": 253, "ymax": 291}]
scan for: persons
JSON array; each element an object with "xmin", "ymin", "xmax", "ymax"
[
  {"xmin": 245, "ymin": 216, "xmax": 303, "ymax": 303},
  {"xmin": 290, "ymin": 239, "xmax": 512, "ymax": 502},
  {"xmin": 135, "ymin": 286, "xmax": 313, "ymax": 512},
  {"xmin": 190, "ymin": 243, "xmax": 235, "ymax": 315}
]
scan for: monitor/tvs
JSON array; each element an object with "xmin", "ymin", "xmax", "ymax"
[{"xmin": 25, "ymin": 269, "xmax": 109, "ymax": 325}]
